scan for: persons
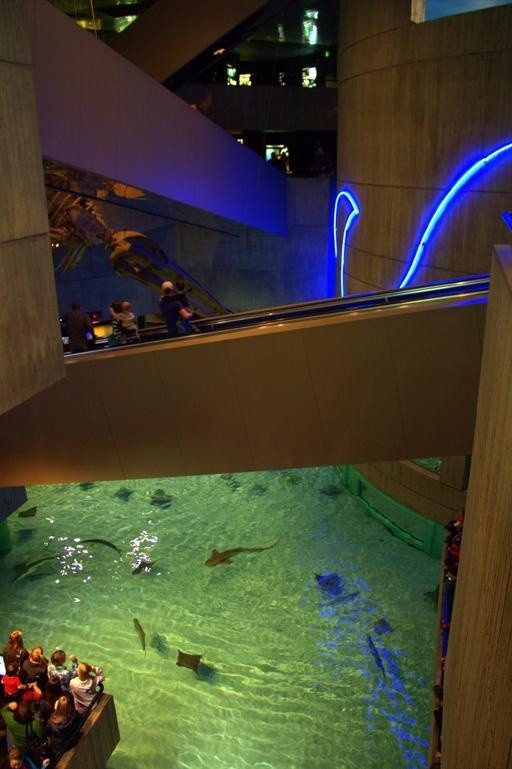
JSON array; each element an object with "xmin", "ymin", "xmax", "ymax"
[
  {"xmin": 160, "ymin": 277, "xmax": 190, "ymax": 333},
  {"xmin": 158, "ymin": 281, "xmax": 194, "ymax": 338},
  {"xmin": 428, "ymin": 505, "xmax": 466, "ymax": 769},
  {"xmin": 107, "ymin": 300, "xmax": 137, "ymax": 336},
  {"xmin": 1, "ymin": 629, "xmax": 107, "ymax": 768},
  {"xmin": 110, "ymin": 300, "xmax": 143, "ymax": 346},
  {"xmin": 64, "ymin": 300, "xmax": 93, "ymax": 353}
]
